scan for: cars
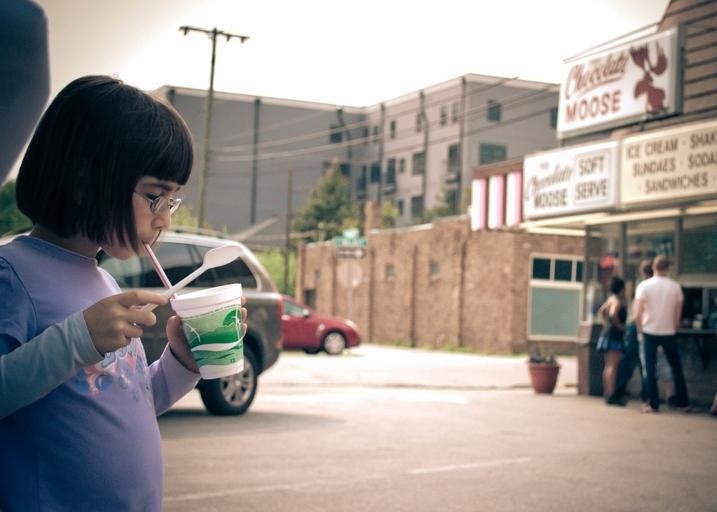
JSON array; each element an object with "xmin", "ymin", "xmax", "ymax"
[
  {"xmin": 97, "ymin": 232, "xmax": 284, "ymax": 416},
  {"xmin": 282, "ymin": 293, "xmax": 363, "ymax": 358}
]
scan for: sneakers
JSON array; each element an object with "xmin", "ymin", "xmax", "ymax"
[{"xmin": 600, "ymin": 391, "xmax": 693, "ymax": 414}]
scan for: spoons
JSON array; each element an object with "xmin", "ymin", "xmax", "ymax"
[{"xmin": 140, "ymin": 243, "xmax": 242, "ymax": 312}]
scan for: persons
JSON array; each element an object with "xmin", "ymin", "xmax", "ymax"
[
  {"xmin": 632, "ymin": 253, "xmax": 691, "ymax": 412},
  {"xmin": 597, "ymin": 274, "xmax": 627, "ymax": 403},
  {"xmin": 605, "ymin": 259, "xmax": 657, "ymax": 406},
  {"xmin": 589, "ymin": 257, "xmax": 623, "ymax": 397},
  {"xmin": 0, "ymin": 72, "xmax": 247, "ymax": 510}
]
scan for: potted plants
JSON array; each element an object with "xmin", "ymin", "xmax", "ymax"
[{"xmin": 527, "ymin": 342, "xmax": 559, "ymax": 396}]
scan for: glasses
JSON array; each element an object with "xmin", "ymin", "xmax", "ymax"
[{"xmin": 132, "ymin": 188, "xmax": 183, "ymax": 217}]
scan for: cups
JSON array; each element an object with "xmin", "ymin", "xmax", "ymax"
[{"xmin": 167, "ymin": 282, "xmax": 246, "ymax": 381}]
